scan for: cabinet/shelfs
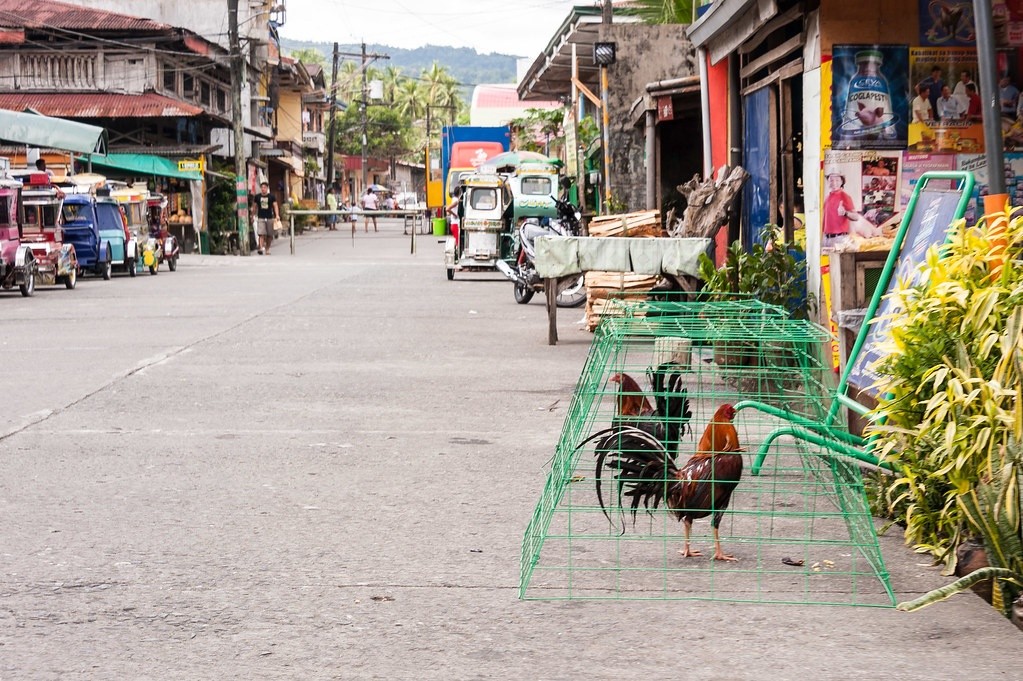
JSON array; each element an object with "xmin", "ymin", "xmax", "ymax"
[{"xmin": 828, "ymin": 248, "xmax": 902, "ymax": 434}]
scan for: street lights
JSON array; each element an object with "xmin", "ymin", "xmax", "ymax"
[{"xmin": 227, "ymin": 4, "xmax": 287, "ymax": 256}]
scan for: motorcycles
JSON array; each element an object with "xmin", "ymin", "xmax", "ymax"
[
  {"xmin": 494, "ymin": 177, "xmax": 587, "ymax": 308},
  {"xmin": 437, "ymin": 151, "xmax": 578, "ymax": 280},
  {"xmin": 0, "ymin": 157, "xmax": 179, "ymax": 297}
]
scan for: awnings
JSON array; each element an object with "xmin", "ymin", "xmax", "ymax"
[
  {"xmin": 77, "ymin": 154, "xmax": 205, "ymax": 180},
  {"xmin": 260, "ymin": 148, "xmax": 291, "ymax": 157},
  {"xmin": 204, "ymin": 170, "xmax": 232, "ymax": 193}
]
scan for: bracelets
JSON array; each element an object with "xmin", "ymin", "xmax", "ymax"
[{"xmin": 844, "ymin": 212, "xmax": 848, "ymax": 217}]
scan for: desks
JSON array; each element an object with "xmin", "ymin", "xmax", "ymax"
[
  {"xmin": 533, "ymin": 234, "xmax": 713, "ymax": 344},
  {"xmin": 170, "ymin": 222, "xmax": 192, "ymax": 253}
]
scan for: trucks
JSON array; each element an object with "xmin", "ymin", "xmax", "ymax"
[{"xmin": 441, "ymin": 126, "xmax": 511, "ymax": 234}]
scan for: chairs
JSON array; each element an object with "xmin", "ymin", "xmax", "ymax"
[{"xmin": 479, "ymin": 195, "xmax": 492, "ymax": 203}]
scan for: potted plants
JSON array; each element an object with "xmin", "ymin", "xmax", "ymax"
[
  {"xmin": 694, "ymin": 239, "xmax": 760, "ymax": 370},
  {"xmin": 576, "ymin": 118, "xmax": 601, "ymax": 160},
  {"xmin": 759, "ymin": 224, "xmax": 818, "ymax": 367},
  {"xmin": 208, "ymin": 180, "xmax": 237, "ymax": 255}
]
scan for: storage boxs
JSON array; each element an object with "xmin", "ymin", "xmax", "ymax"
[
  {"xmin": 30, "ymin": 172, "xmax": 49, "ymax": 184},
  {"xmin": 110, "ymin": 180, "xmax": 150, "ymax": 202}
]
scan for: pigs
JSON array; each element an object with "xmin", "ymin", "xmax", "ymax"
[{"xmin": 854, "ymin": 102, "xmax": 883, "ymax": 129}]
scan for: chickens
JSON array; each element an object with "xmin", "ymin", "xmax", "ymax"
[
  {"xmin": 609, "ymin": 360, "xmax": 692, "ymax": 462},
  {"xmin": 570, "ymin": 403, "xmax": 743, "ymax": 562}
]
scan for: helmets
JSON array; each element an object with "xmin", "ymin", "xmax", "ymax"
[{"xmin": 824, "ymin": 166, "xmax": 846, "ymax": 186}]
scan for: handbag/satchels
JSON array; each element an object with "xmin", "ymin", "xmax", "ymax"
[{"xmin": 273, "ymin": 219, "xmax": 282, "ymax": 230}]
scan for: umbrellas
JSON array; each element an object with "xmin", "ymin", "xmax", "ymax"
[
  {"xmin": 485, "ymin": 151, "xmax": 565, "ymax": 168},
  {"xmin": 370, "ymin": 184, "xmax": 389, "ymax": 191}
]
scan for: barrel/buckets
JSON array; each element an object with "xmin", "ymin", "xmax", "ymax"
[
  {"xmin": 655, "ymin": 336, "xmax": 692, "ymax": 371},
  {"xmin": 432, "ymin": 217, "xmax": 446, "ymax": 236}
]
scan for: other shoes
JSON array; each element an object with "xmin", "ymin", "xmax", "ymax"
[{"xmin": 257, "ymin": 250, "xmax": 263, "ymax": 254}]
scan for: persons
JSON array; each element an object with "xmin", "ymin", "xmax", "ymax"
[
  {"xmin": 863, "ymin": 206, "xmax": 882, "ymax": 224},
  {"xmin": 446, "ymin": 186, "xmax": 461, "ymax": 245},
  {"xmin": 764, "ymin": 193, "xmax": 806, "ymax": 253},
  {"xmin": 863, "ymin": 178, "xmax": 881, "ymax": 191},
  {"xmin": 251, "ymin": 182, "xmax": 279, "ymax": 255},
  {"xmin": 349, "ymin": 202, "xmax": 361, "ymax": 231},
  {"xmin": 912, "ymin": 65, "xmax": 1023, "ymax": 144},
  {"xmin": 363, "ymin": 188, "xmax": 379, "ymax": 232},
  {"xmin": 327, "ymin": 188, "xmax": 338, "ymax": 231},
  {"xmin": 66, "ymin": 161, "xmax": 82, "ymax": 176},
  {"xmin": 386, "ymin": 196, "xmax": 398, "ymax": 217},
  {"xmin": 866, "ymin": 160, "xmax": 889, "ymax": 175},
  {"xmin": 36, "ymin": 159, "xmax": 54, "ymax": 176},
  {"xmin": 824, "ymin": 169, "xmax": 859, "ymax": 238}
]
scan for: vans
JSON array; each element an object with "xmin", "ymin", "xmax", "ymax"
[{"xmin": 397, "ymin": 193, "xmax": 418, "ymax": 218}]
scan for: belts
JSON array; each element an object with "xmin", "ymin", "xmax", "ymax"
[{"xmin": 826, "ymin": 232, "xmax": 845, "ymax": 238}]
